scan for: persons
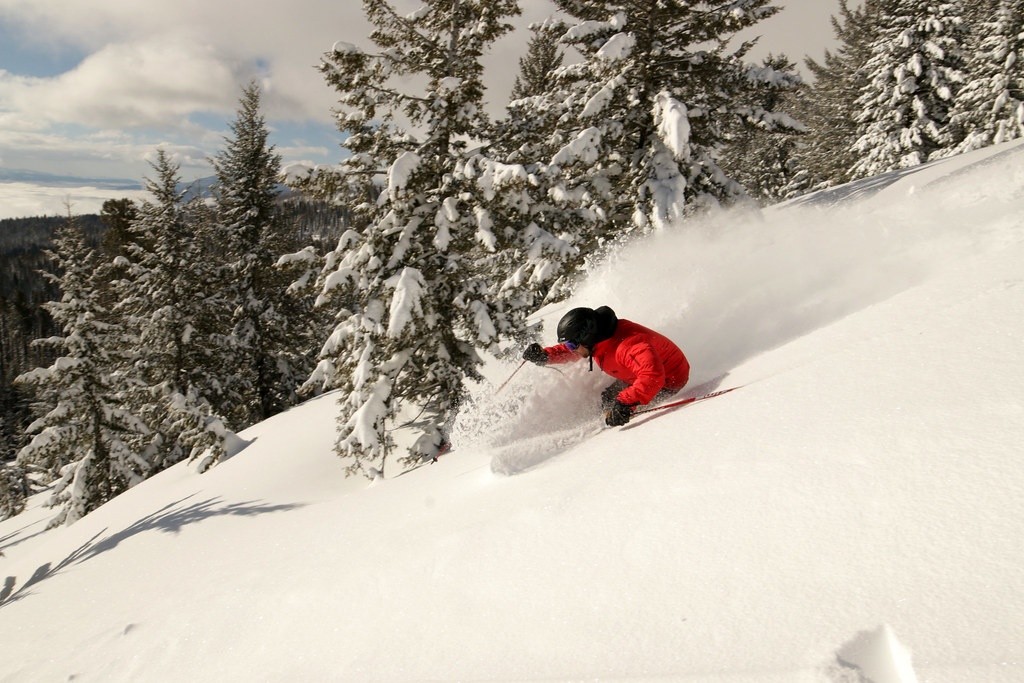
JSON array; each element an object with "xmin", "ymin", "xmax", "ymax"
[{"xmin": 522, "ymin": 306, "xmax": 690, "ymax": 426}]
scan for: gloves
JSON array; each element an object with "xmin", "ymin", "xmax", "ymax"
[
  {"xmin": 522, "ymin": 343, "xmax": 547, "ymax": 367},
  {"xmin": 605, "ymin": 402, "xmax": 630, "ymax": 426}
]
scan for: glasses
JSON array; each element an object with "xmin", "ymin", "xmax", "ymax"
[{"xmin": 565, "ymin": 338, "xmax": 578, "ymax": 351}]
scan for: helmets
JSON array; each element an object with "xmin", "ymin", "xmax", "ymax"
[{"xmin": 557, "ymin": 308, "xmax": 595, "ymax": 343}]
